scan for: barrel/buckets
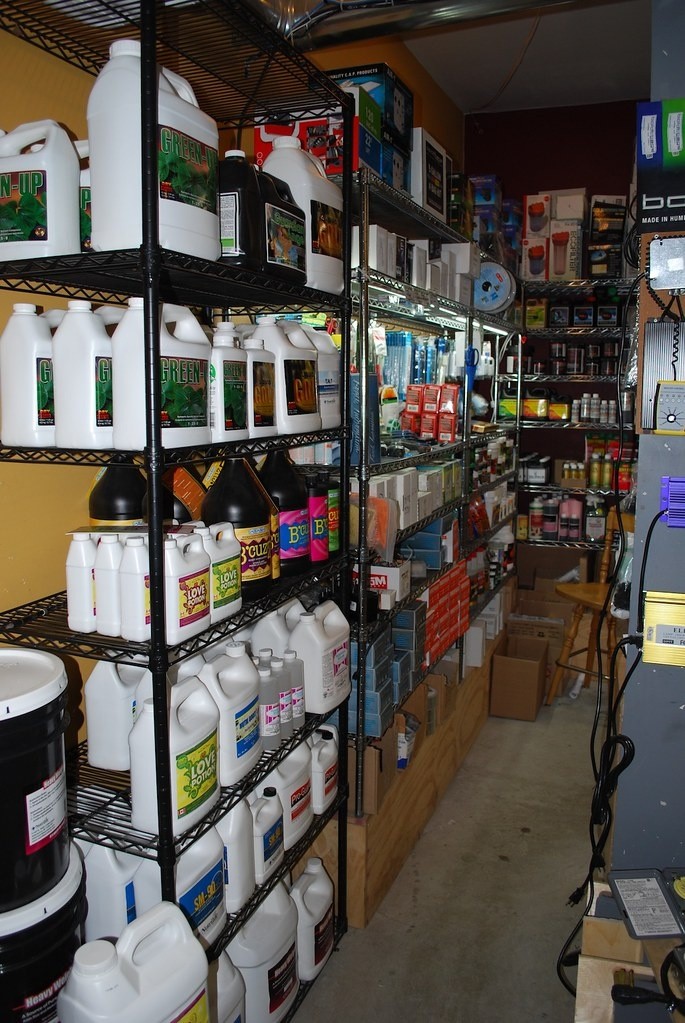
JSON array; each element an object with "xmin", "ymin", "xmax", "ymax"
[
  {"xmin": 0, "ymin": 644, "xmax": 89, "ymax": 1023},
  {"xmin": 82, "ymin": 597, "xmax": 352, "ymax": 840},
  {"xmin": 63, "ymin": 518, "xmax": 242, "ymax": 647},
  {"xmin": 54, "ymin": 720, "xmax": 339, "ymax": 1023},
  {"xmin": 0, "ymin": 296, "xmax": 342, "ymax": 449},
  {"xmin": 1, "ymin": 39, "xmax": 349, "ymax": 295}
]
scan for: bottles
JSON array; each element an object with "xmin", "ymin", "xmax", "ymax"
[
  {"xmin": 250, "ymin": 657, "xmax": 260, "ymax": 671},
  {"xmin": 88, "ymin": 445, "xmax": 345, "ymax": 596},
  {"xmin": 281, "ymin": 650, "xmax": 306, "ymax": 730},
  {"xmin": 258, "ymin": 669, "xmax": 281, "ymax": 750},
  {"xmin": 270, "ymin": 659, "xmax": 293, "ymax": 739},
  {"xmin": 465, "ymin": 391, "xmax": 639, "ymax": 606},
  {"xmin": 259, "ymin": 648, "xmax": 278, "ymax": 670}
]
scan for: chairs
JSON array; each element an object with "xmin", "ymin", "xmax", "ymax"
[{"xmin": 544, "ymin": 507, "xmax": 637, "ymax": 707}]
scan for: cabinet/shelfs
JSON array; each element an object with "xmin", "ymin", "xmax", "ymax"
[
  {"xmin": 1, "ymin": 1, "xmax": 356, "ymax": 1023},
  {"xmin": 326, "ymin": 167, "xmax": 645, "ymax": 930}
]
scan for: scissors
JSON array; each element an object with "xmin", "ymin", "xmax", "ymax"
[
  {"xmin": 466, "ymin": 346, "xmax": 479, "ymax": 391},
  {"xmin": 326, "ymin": 317, "xmax": 338, "ymax": 335}
]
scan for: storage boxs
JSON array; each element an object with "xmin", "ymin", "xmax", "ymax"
[{"xmin": 327, "ymin": 65, "xmax": 629, "ymax": 814}]
